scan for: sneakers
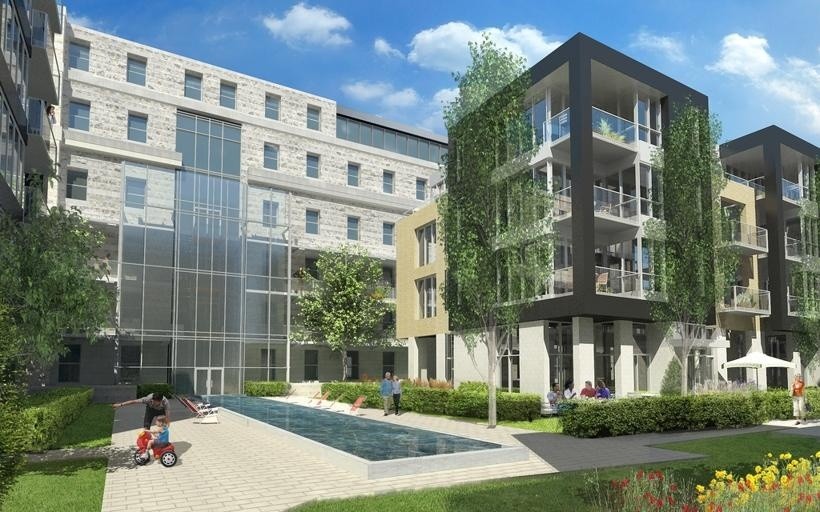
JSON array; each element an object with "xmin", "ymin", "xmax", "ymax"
[{"xmin": 141, "ymin": 453, "xmax": 150, "ymax": 461}]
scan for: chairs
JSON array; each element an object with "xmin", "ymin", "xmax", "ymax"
[
  {"xmin": 303, "ymin": 391, "xmax": 371, "ymax": 420},
  {"xmin": 168, "ymin": 392, "xmax": 219, "ymax": 425}
]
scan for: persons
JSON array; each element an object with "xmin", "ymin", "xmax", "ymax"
[
  {"xmin": 547, "ymin": 379, "xmax": 610, "ymax": 407},
  {"xmin": 381, "ymin": 372, "xmax": 394, "ymax": 416},
  {"xmin": 790, "ymin": 372, "xmax": 806, "ymax": 424},
  {"xmin": 139, "ymin": 416, "xmax": 169, "ymax": 461},
  {"xmin": 109, "ymin": 392, "xmax": 172, "ymax": 429},
  {"xmin": 393, "ymin": 374, "xmax": 401, "ymax": 416}
]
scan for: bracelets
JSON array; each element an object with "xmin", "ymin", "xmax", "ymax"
[{"xmin": 121, "ymin": 403, "xmax": 123, "ymax": 407}]
